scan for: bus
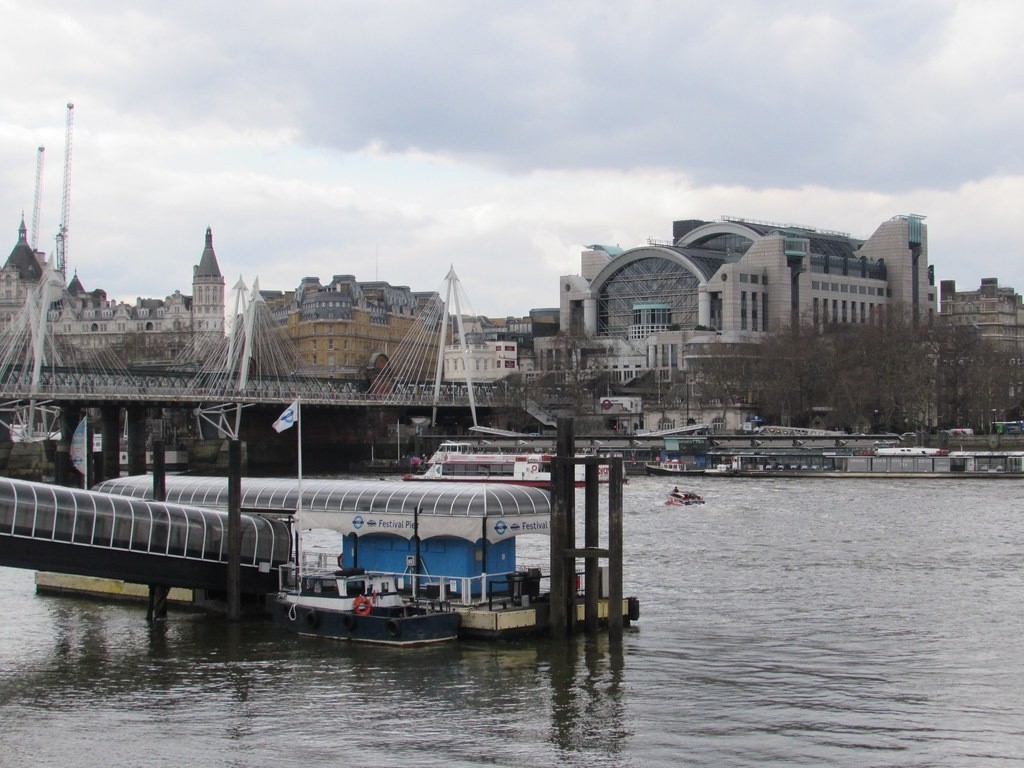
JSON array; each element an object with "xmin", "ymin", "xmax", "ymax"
[{"xmin": 988, "ymin": 420, "xmax": 1023, "ymax": 434}]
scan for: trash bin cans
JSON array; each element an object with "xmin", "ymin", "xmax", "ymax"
[{"xmin": 507, "ymin": 568, "xmax": 542, "ymax": 606}]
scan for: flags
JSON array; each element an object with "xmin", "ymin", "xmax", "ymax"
[
  {"xmin": 411, "ymin": 457, "xmax": 420, "ymax": 464},
  {"xmin": 272, "ymin": 399, "xmax": 298, "ymax": 433}
]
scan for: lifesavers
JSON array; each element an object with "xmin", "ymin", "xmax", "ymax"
[
  {"xmin": 531, "ymin": 465, "xmax": 537, "ymax": 472},
  {"xmin": 603, "ymin": 399, "xmax": 612, "ymax": 410},
  {"xmin": 352, "ymin": 594, "xmax": 372, "ymax": 618}
]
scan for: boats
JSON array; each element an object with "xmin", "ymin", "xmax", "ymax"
[
  {"xmin": 644, "ymin": 446, "xmax": 1024, "ymax": 478},
  {"xmin": 403, "ymin": 440, "xmax": 630, "ymax": 488},
  {"xmin": 665, "ymin": 491, "xmax": 706, "ymax": 505},
  {"xmin": 35, "ymin": 472, "xmax": 639, "ymax": 643}
]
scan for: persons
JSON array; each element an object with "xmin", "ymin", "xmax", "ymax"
[{"xmin": 673, "ymin": 487, "xmax": 678, "ymax": 492}]
